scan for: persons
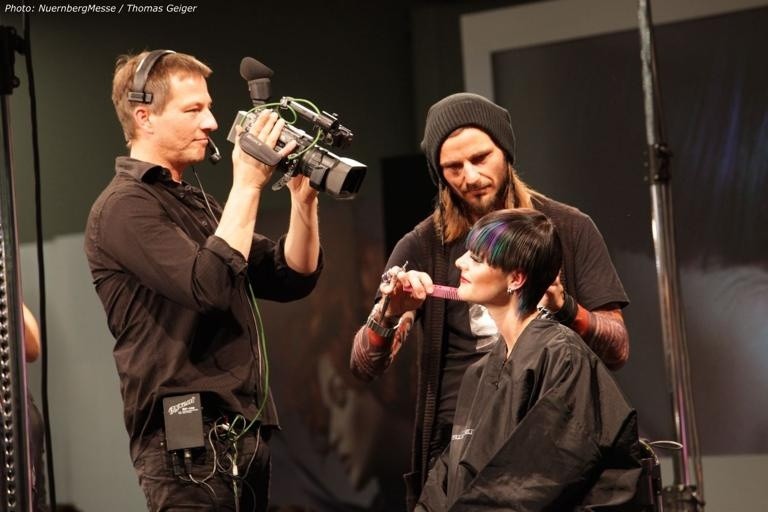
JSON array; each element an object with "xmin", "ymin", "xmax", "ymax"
[
  {"xmin": 21, "ymin": 299, "xmax": 47, "ymax": 512},
  {"xmin": 345, "ymin": 92, "xmax": 631, "ymax": 512},
  {"xmin": 411, "ymin": 206, "xmax": 649, "ymax": 512},
  {"xmin": 81, "ymin": 49, "xmax": 328, "ymax": 512}
]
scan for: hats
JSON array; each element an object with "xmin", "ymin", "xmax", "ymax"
[{"xmin": 421, "ymin": 90, "xmax": 515, "ymax": 183}]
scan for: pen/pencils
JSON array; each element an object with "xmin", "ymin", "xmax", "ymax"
[{"xmin": 376, "ymin": 260, "xmax": 410, "ymax": 326}]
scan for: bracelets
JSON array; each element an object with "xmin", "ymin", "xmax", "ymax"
[
  {"xmin": 552, "ymin": 293, "xmax": 579, "ymax": 328},
  {"xmin": 367, "ymin": 302, "xmax": 403, "ymax": 339}
]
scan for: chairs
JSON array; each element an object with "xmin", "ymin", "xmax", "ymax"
[{"xmin": 601, "ymin": 438, "xmax": 664, "ymax": 512}]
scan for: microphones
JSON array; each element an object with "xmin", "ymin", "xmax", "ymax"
[{"xmin": 208, "ymin": 137, "xmax": 222, "ymax": 164}]
